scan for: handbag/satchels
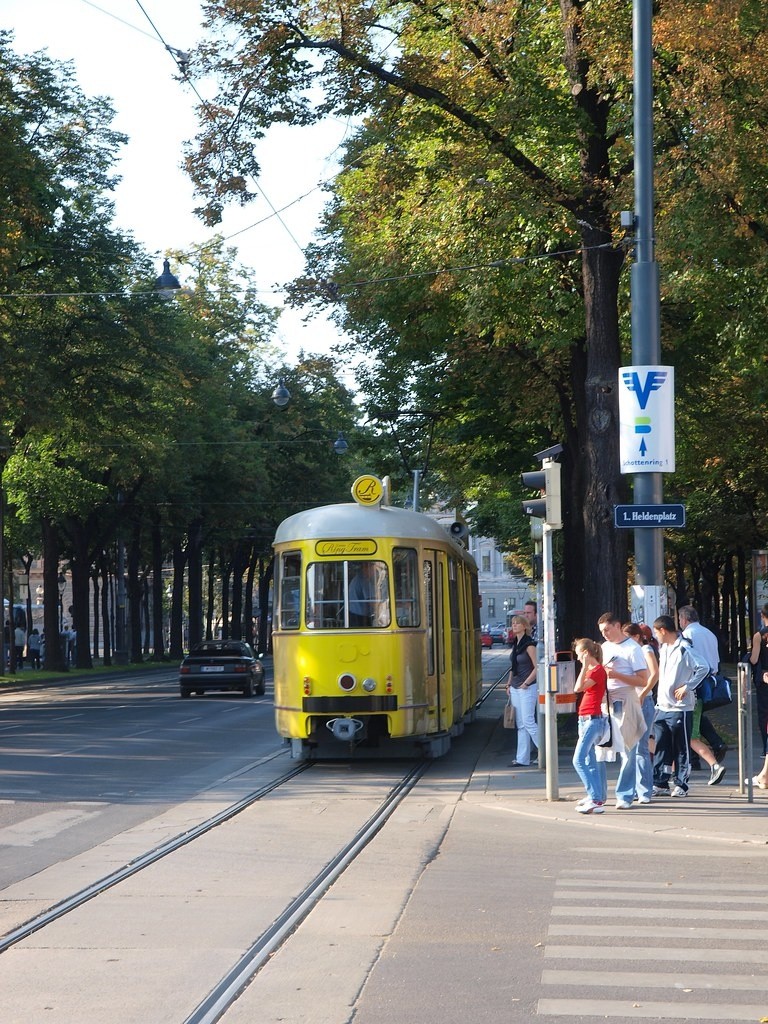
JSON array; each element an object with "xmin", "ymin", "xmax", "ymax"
[
  {"xmin": 698, "ymin": 675, "xmax": 732, "ymax": 711},
  {"xmin": 503, "ymin": 704, "xmax": 516, "ymax": 730}
]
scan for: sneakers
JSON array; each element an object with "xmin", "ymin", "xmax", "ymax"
[
  {"xmin": 671, "ymin": 785, "xmax": 688, "ymax": 798},
  {"xmin": 707, "ymin": 763, "xmax": 727, "ymax": 785},
  {"xmin": 651, "ymin": 785, "xmax": 672, "ymax": 796}
]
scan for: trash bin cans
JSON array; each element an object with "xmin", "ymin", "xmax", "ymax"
[{"xmin": 538, "ymin": 650, "xmax": 576, "ymax": 714}]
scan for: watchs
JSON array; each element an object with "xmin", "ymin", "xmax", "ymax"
[{"xmin": 506, "ymin": 684, "xmax": 511, "ymax": 689}]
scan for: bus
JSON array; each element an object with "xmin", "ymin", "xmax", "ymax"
[{"xmin": 268, "ymin": 469, "xmax": 485, "ymax": 763}]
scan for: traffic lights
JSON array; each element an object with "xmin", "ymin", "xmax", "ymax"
[{"xmin": 518, "ymin": 442, "xmax": 568, "ymax": 537}]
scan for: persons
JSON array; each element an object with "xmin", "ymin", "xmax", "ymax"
[
  {"xmin": 572, "ymin": 638, "xmax": 607, "ymax": 814},
  {"xmin": 578, "ymin": 611, "xmax": 648, "ymax": 808},
  {"xmin": 3, "ymin": 619, "xmax": 76, "ymax": 671},
  {"xmin": 166, "ymin": 630, "xmax": 170, "ymax": 648},
  {"xmin": 621, "ymin": 605, "xmax": 728, "ymax": 801},
  {"xmin": 505, "ymin": 600, "xmax": 544, "ymax": 767},
  {"xmin": 651, "ymin": 615, "xmax": 710, "ymax": 797},
  {"xmin": 744, "ymin": 602, "xmax": 768, "ymax": 789},
  {"xmin": 348, "ymin": 561, "xmax": 383, "ymax": 627}
]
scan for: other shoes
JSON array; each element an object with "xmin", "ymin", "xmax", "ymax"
[
  {"xmin": 575, "ymin": 797, "xmax": 605, "ymax": 814},
  {"xmin": 745, "ymin": 774, "xmax": 767, "ymax": 789},
  {"xmin": 617, "ymin": 798, "xmax": 633, "ymax": 810},
  {"xmin": 639, "ymin": 797, "xmax": 651, "ymax": 804},
  {"xmin": 506, "ymin": 760, "xmax": 530, "ymax": 767},
  {"xmin": 714, "ymin": 742, "xmax": 728, "ymax": 763}
]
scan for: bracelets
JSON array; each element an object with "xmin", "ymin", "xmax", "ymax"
[{"xmin": 524, "ymin": 681, "xmax": 530, "ymax": 686}]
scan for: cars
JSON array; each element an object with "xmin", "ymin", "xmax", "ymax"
[
  {"xmin": 177, "ymin": 639, "xmax": 266, "ymax": 699},
  {"xmin": 480, "ymin": 624, "xmax": 509, "ymax": 650}
]
